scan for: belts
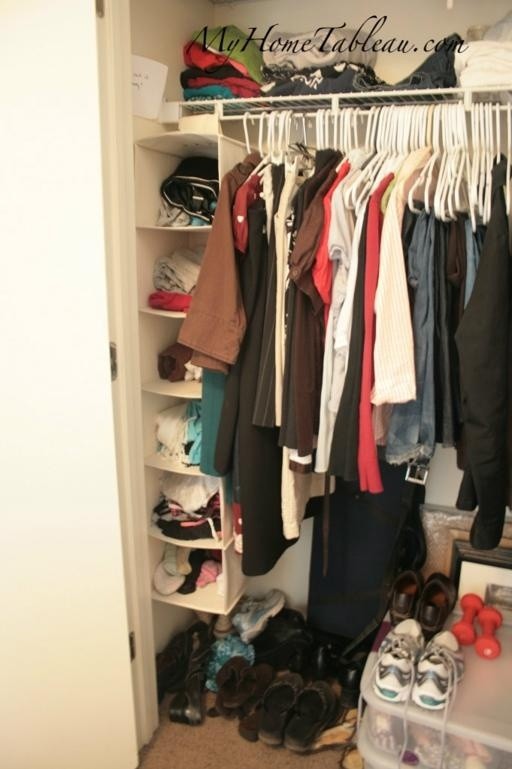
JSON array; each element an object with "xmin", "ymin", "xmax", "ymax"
[{"xmin": 405, "ymin": 453, "xmax": 429, "ymax": 485}]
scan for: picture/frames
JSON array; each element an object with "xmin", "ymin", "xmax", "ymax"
[{"xmin": 451, "ymin": 537, "xmax": 512, "ymax": 626}]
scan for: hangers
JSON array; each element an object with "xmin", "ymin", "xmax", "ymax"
[{"xmin": 221, "ymin": 103, "xmax": 512, "ymax": 234}]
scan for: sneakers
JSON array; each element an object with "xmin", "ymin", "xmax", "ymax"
[
  {"xmin": 374, "ymin": 618, "xmax": 424, "ymax": 704},
  {"xmin": 412, "ymin": 630, "xmax": 464, "ymax": 709}
]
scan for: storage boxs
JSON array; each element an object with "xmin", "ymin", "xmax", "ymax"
[
  {"xmin": 357, "ymin": 611, "xmax": 512, "ymax": 769},
  {"xmin": 357, "ymin": 708, "xmax": 420, "ymax": 769}
]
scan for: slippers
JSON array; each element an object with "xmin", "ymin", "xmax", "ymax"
[{"xmin": 159, "ymin": 589, "xmax": 361, "ymax": 754}]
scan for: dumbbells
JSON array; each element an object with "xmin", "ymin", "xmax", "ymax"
[
  {"xmin": 474, "ymin": 606, "xmax": 503, "ymax": 660},
  {"xmin": 452, "ymin": 593, "xmax": 486, "ymax": 646}
]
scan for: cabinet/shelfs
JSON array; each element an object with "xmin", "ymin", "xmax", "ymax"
[{"xmin": 136, "ymin": 134, "xmax": 268, "ymax": 618}]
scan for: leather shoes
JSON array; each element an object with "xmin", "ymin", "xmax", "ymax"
[
  {"xmin": 388, "ymin": 570, "xmax": 424, "ymax": 627},
  {"xmin": 420, "ymin": 571, "xmax": 458, "ymax": 638}
]
